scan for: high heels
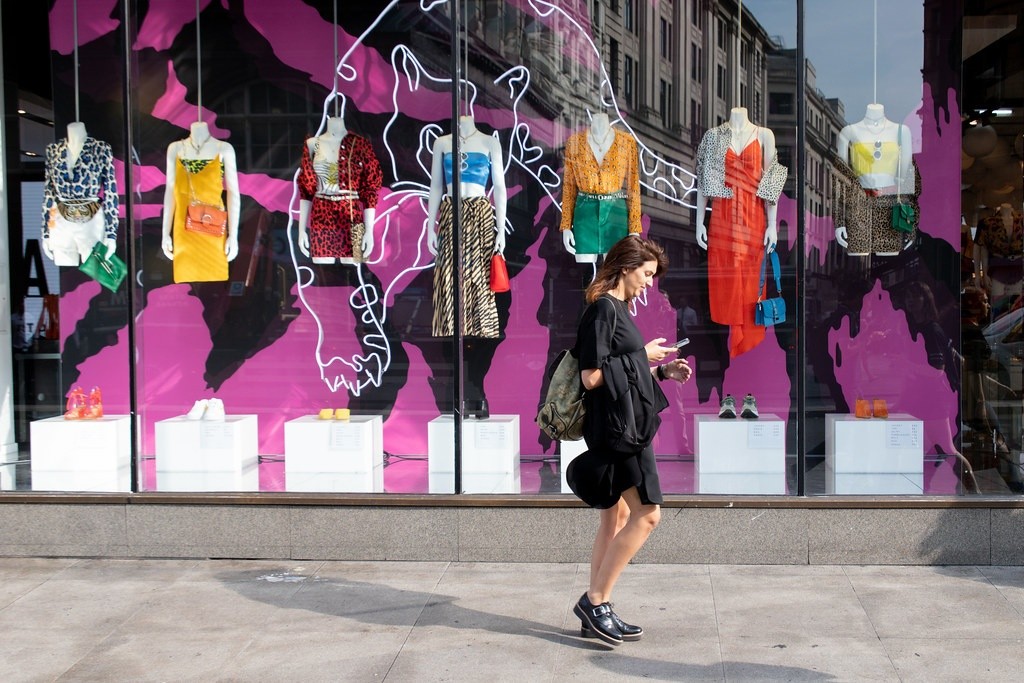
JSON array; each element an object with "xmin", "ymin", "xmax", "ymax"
[
  {"xmin": 83, "ymin": 386, "xmax": 103, "ymax": 420},
  {"xmin": 64, "ymin": 386, "xmax": 88, "ymax": 420}
]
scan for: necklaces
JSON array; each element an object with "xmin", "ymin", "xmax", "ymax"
[
  {"xmin": 862, "ymin": 115, "xmax": 887, "ymax": 135},
  {"xmin": 590, "ymin": 128, "xmax": 611, "ymax": 152},
  {"xmin": 324, "ymin": 129, "xmax": 346, "ymax": 136},
  {"xmin": 190, "ymin": 134, "xmax": 212, "ymax": 154},
  {"xmin": 460, "ymin": 129, "xmax": 478, "ymax": 143},
  {"xmin": 734, "ymin": 121, "xmax": 749, "ymax": 140}
]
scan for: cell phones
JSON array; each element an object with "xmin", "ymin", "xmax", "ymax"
[{"xmin": 669, "ymin": 338, "xmax": 689, "ymax": 349}]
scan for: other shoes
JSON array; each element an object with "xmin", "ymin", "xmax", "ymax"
[
  {"xmin": 335, "ymin": 409, "xmax": 350, "ymax": 419},
  {"xmin": 204, "ymin": 397, "xmax": 225, "ymax": 423},
  {"xmin": 186, "ymin": 399, "xmax": 209, "ymax": 421},
  {"xmin": 856, "ymin": 399, "xmax": 871, "ymax": 418},
  {"xmin": 320, "ymin": 408, "xmax": 334, "ymax": 419},
  {"xmin": 873, "ymin": 400, "xmax": 888, "ymax": 418}
]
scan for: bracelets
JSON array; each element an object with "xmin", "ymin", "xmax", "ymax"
[{"xmin": 657, "ymin": 364, "xmax": 669, "ymax": 381}]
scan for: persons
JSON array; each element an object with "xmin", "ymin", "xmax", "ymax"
[
  {"xmin": 835, "ymin": 103, "xmax": 917, "ymax": 257},
  {"xmin": 299, "ymin": 117, "xmax": 382, "ymax": 264},
  {"xmin": 428, "ymin": 115, "xmax": 508, "ymax": 338},
  {"xmin": 677, "ymin": 294, "xmax": 696, "ymax": 325},
  {"xmin": 791, "ymin": 269, "xmax": 1024, "ymax": 495},
  {"xmin": 40, "ymin": 122, "xmax": 119, "ymax": 267},
  {"xmin": 961, "ymin": 203, "xmax": 1024, "ymax": 333},
  {"xmin": 696, "ymin": 107, "xmax": 787, "ymax": 359},
  {"xmin": 161, "ymin": 122, "xmax": 241, "ymax": 283},
  {"xmin": 560, "ymin": 114, "xmax": 644, "ymax": 263},
  {"xmin": 566, "ymin": 235, "xmax": 692, "ymax": 647}
]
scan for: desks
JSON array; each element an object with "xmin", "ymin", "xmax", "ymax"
[{"xmin": 15, "ymin": 350, "xmax": 86, "ymax": 441}]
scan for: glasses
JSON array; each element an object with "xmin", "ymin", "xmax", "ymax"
[
  {"xmin": 460, "ymin": 152, "xmax": 468, "ymax": 172},
  {"xmin": 874, "ymin": 140, "xmax": 882, "ymax": 160}
]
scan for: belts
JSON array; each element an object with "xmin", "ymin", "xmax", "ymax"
[{"xmin": 578, "ymin": 192, "xmax": 628, "ymax": 201}]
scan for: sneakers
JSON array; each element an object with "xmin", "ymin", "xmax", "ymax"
[
  {"xmin": 719, "ymin": 394, "xmax": 736, "ymax": 418},
  {"xmin": 740, "ymin": 393, "xmax": 759, "ymax": 418}
]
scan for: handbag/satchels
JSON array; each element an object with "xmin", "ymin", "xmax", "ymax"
[
  {"xmin": 490, "ymin": 255, "xmax": 512, "ymax": 292},
  {"xmin": 185, "ymin": 203, "xmax": 227, "ymax": 236},
  {"xmin": 79, "ymin": 241, "xmax": 129, "ymax": 293},
  {"xmin": 534, "ymin": 296, "xmax": 617, "ymax": 441},
  {"xmin": 351, "ymin": 222, "xmax": 369, "ymax": 263},
  {"xmin": 891, "ymin": 204, "xmax": 914, "ymax": 233},
  {"xmin": 54, "ymin": 197, "xmax": 101, "ymax": 223},
  {"xmin": 755, "ymin": 297, "xmax": 786, "ymax": 327}
]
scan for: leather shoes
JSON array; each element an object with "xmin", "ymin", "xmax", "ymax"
[
  {"xmin": 476, "ymin": 400, "xmax": 488, "ymax": 418},
  {"xmin": 581, "ymin": 602, "xmax": 644, "ymax": 641},
  {"xmin": 573, "ymin": 592, "xmax": 624, "ymax": 646},
  {"xmin": 462, "ymin": 400, "xmax": 469, "ymax": 418}
]
